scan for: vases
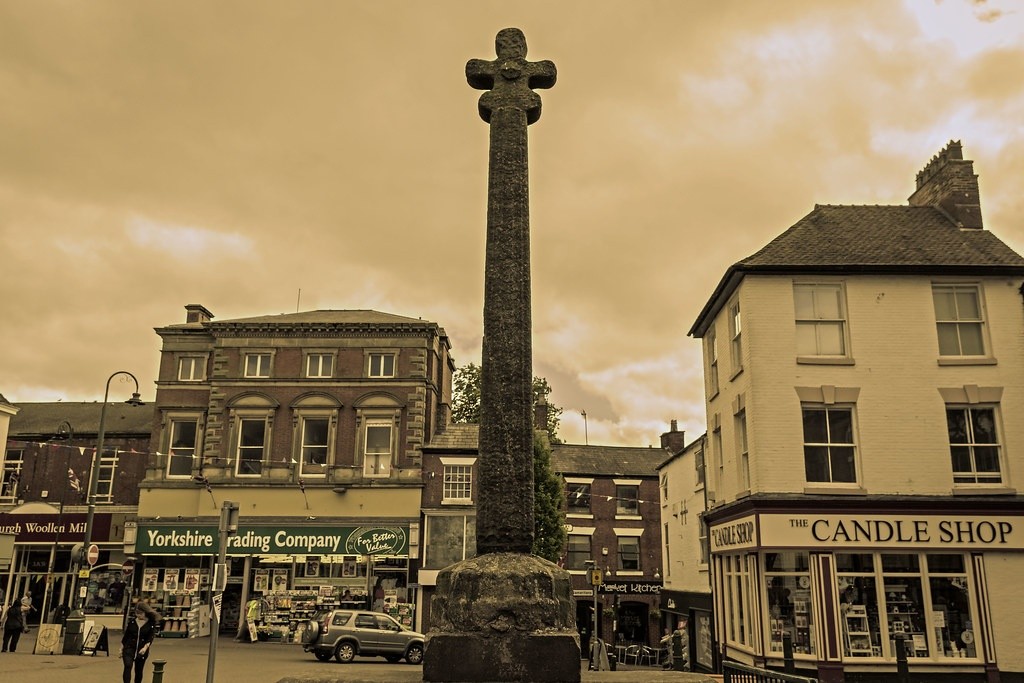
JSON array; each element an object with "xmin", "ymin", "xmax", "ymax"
[{"xmin": 257, "ymin": 632, "xmax": 269, "ymax": 641}]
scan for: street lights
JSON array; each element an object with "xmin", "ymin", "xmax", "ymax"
[
  {"xmin": 75, "ymin": 371, "xmax": 144, "ymax": 610},
  {"xmin": 581, "ymin": 409, "xmax": 588, "ymax": 444},
  {"xmin": 584, "ymin": 558, "xmax": 601, "ymax": 669},
  {"xmin": 40, "ymin": 419, "xmax": 75, "ymax": 624}
]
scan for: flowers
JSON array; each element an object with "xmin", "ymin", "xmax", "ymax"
[{"xmin": 256, "ymin": 629, "xmax": 273, "ymax": 635}]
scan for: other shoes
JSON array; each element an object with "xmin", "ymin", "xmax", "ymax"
[
  {"xmin": 1, "ymin": 649, "xmax": 6, "ymax": 652},
  {"xmin": 25, "ymin": 629, "xmax": 30, "ymax": 633},
  {"xmin": 10, "ymin": 650, "xmax": 15, "ymax": 652}
]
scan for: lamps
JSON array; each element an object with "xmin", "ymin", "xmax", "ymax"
[
  {"xmin": 605, "ymin": 566, "xmax": 611, "ymax": 576},
  {"xmin": 653, "ymin": 568, "xmax": 660, "ymax": 578}
]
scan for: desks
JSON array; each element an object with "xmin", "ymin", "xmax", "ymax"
[{"xmin": 651, "ymin": 648, "xmax": 665, "ymax": 666}]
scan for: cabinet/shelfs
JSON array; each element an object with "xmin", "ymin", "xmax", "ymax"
[
  {"xmin": 388, "ymin": 603, "xmax": 412, "ymax": 632},
  {"xmin": 128, "ymin": 568, "xmax": 209, "ymax": 637},
  {"xmin": 764, "ymin": 585, "xmax": 950, "ymax": 657},
  {"xmin": 255, "ymin": 590, "xmax": 340, "ymax": 644}
]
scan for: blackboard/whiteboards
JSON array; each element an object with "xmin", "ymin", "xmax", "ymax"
[{"xmin": 79, "ymin": 626, "xmax": 108, "ymax": 652}]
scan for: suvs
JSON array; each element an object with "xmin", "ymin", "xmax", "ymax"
[{"xmin": 302, "ymin": 609, "xmax": 426, "ymax": 665}]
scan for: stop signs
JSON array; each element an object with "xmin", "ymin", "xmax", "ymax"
[
  {"xmin": 122, "ymin": 560, "xmax": 134, "ymax": 575},
  {"xmin": 87, "ymin": 545, "xmax": 99, "ymax": 566}
]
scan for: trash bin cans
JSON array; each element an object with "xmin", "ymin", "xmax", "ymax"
[{"xmin": 53, "ymin": 604, "xmax": 70, "ymax": 627}]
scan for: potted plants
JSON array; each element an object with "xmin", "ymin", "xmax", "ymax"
[
  {"xmin": 651, "ymin": 609, "xmax": 660, "ymax": 619},
  {"xmin": 150, "ymin": 595, "xmax": 157, "ymax": 603},
  {"xmin": 143, "ymin": 595, "xmax": 149, "ymax": 603},
  {"xmin": 603, "ymin": 609, "xmax": 614, "ymax": 617},
  {"xmin": 132, "ymin": 596, "xmax": 139, "ymax": 603}
]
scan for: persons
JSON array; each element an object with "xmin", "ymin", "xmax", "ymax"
[
  {"xmin": 1, "ymin": 600, "xmax": 23, "ymax": 652},
  {"xmin": 87, "ymin": 590, "xmax": 106, "ymax": 613},
  {"xmin": 375, "ymin": 584, "xmax": 385, "ymax": 598},
  {"xmin": 775, "ymin": 588, "xmax": 791, "ymax": 621},
  {"xmin": 108, "ymin": 572, "xmax": 128, "ymax": 606},
  {"xmin": 396, "ymin": 584, "xmax": 407, "ymax": 602},
  {"xmin": 840, "ymin": 585, "xmax": 854, "ymax": 604},
  {"xmin": 13, "ymin": 590, "xmax": 39, "ymax": 634},
  {"xmin": 342, "ymin": 590, "xmax": 353, "ymax": 601},
  {"xmin": 117, "ymin": 602, "xmax": 158, "ymax": 683}
]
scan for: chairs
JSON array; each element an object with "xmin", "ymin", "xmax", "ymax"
[{"xmin": 605, "ymin": 641, "xmax": 656, "ymax": 667}]
